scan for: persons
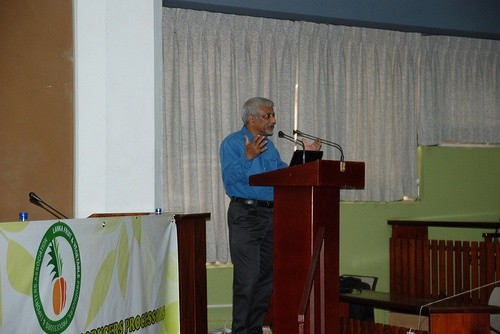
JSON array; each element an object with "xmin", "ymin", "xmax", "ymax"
[{"xmin": 221, "ymin": 97, "xmax": 321, "ymax": 334}]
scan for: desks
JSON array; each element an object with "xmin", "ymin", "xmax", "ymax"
[
  {"xmin": 340, "ymin": 290, "xmax": 500, "ymax": 334},
  {"xmin": 387, "ymin": 219, "xmax": 499, "ymax": 303},
  {"xmin": 0, "ymin": 213, "xmax": 211, "ymax": 334}
]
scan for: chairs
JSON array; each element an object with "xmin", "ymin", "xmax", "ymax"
[{"xmin": 487, "ymin": 287, "xmax": 500, "ymax": 334}]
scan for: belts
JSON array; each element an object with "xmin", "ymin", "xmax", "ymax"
[{"xmin": 230, "ymin": 196, "xmax": 274, "ymax": 209}]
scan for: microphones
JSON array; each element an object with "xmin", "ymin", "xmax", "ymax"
[
  {"xmin": 29, "ymin": 192, "xmax": 69, "ymax": 219},
  {"xmin": 293, "ymin": 130, "xmax": 344, "ymax": 163},
  {"xmin": 278, "ymin": 131, "xmax": 305, "ymax": 164}
]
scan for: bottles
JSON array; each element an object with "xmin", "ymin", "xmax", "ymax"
[{"xmin": 18, "ymin": 212, "xmax": 29, "ymax": 223}]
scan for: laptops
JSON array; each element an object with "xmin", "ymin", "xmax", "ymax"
[{"xmin": 289, "ymin": 150, "xmax": 323, "ymax": 166}]
